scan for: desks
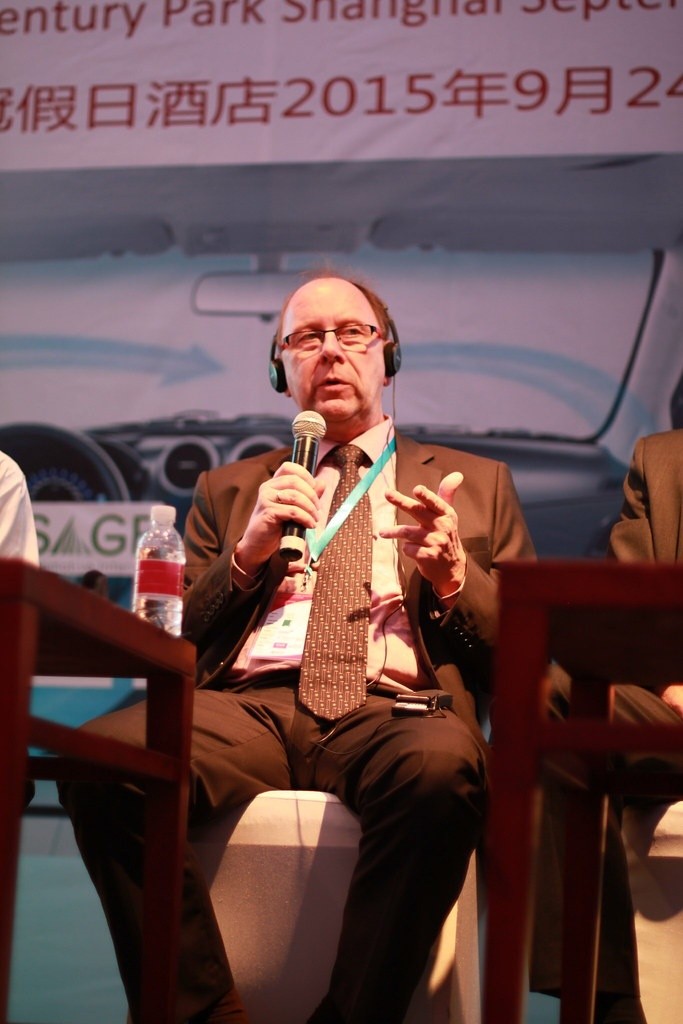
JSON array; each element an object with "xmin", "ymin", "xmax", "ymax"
[
  {"xmin": 478, "ymin": 560, "xmax": 683, "ymax": 1024},
  {"xmin": 0, "ymin": 554, "xmax": 193, "ymax": 1024}
]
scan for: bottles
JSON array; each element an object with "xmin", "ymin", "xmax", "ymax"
[{"xmin": 132, "ymin": 504, "xmax": 187, "ymax": 636}]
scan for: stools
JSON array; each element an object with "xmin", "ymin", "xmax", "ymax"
[{"xmin": 208, "ymin": 788, "xmax": 458, "ymax": 1023}]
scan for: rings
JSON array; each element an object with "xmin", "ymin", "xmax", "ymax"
[{"xmin": 275, "ymin": 490, "xmax": 281, "ymax": 503}]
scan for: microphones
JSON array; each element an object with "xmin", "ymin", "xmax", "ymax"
[{"xmin": 280, "ymin": 411, "xmax": 327, "ymax": 562}]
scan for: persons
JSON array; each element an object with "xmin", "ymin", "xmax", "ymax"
[
  {"xmin": 546, "ymin": 426, "xmax": 683, "ymax": 859},
  {"xmin": 0, "ymin": 451, "xmax": 39, "ymax": 567},
  {"xmin": 55, "ymin": 276, "xmax": 539, "ymax": 1024}
]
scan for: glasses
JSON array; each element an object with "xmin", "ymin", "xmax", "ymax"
[{"xmin": 282, "ymin": 324, "xmax": 384, "ymax": 350}]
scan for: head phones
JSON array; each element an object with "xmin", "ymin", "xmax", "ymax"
[{"xmin": 270, "ymin": 304, "xmax": 401, "ymax": 392}]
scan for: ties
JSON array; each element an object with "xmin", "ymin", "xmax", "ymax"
[{"xmin": 296, "ymin": 444, "xmax": 372, "ymax": 722}]
